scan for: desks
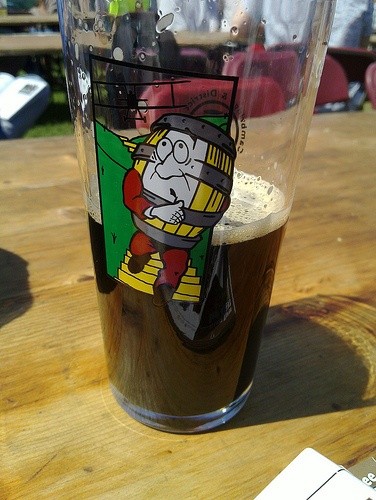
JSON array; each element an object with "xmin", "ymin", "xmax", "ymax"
[
  {"xmin": 0, "ymin": 11, "xmax": 103, "ymax": 30},
  {"xmin": 1, "ymin": 26, "xmax": 234, "ymax": 110},
  {"xmin": 0, "ymin": 107, "xmax": 376, "ymax": 500}
]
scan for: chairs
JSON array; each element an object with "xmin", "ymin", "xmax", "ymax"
[
  {"xmin": 136, "ymin": 78, "xmax": 286, "ymax": 127},
  {"xmin": 222, "ymin": 46, "xmax": 352, "ymax": 113}
]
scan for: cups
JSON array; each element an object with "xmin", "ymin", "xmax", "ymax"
[{"xmin": 54, "ymin": 1, "xmax": 336, "ymax": 433}]
